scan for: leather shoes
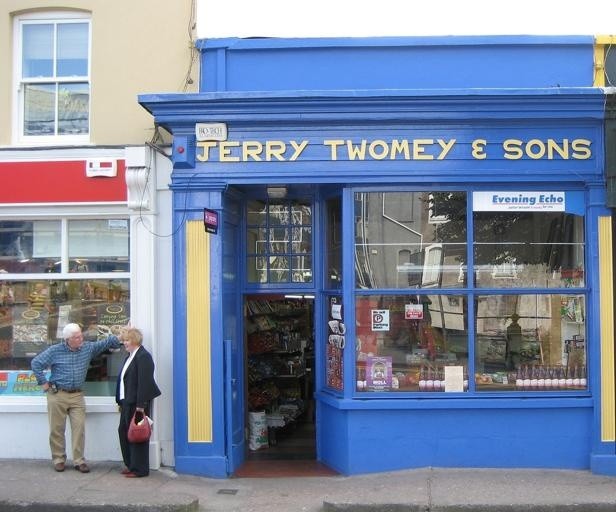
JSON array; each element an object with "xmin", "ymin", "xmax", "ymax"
[
  {"xmin": 55, "ymin": 463, "xmax": 65, "ymax": 471},
  {"xmin": 75, "ymin": 465, "xmax": 89, "ymax": 472},
  {"xmin": 122, "ymin": 468, "xmax": 138, "ymax": 477}
]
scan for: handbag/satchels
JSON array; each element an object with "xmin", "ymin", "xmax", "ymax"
[{"xmin": 127, "ymin": 410, "xmax": 150, "ymax": 442}]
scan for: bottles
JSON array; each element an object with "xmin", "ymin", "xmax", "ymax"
[
  {"xmin": 419, "ymin": 365, "xmax": 445, "ymax": 392},
  {"xmin": 463, "ymin": 365, "xmax": 469, "ymax": 392},
  {"xmin": 516, "ymin": 364, "xmax": 587, "ymax": 390}
]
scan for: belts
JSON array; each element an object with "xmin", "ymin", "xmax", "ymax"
[{"xmin": 60, "ymin": 389, "xmax": 81, "ymax": 393}]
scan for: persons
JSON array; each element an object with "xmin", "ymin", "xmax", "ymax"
[
  {"xmin": 28, "ymin": 321, "xmax": 124, "ymax": 474},
  {"xmin": 115, "ymin": 326, "xmax": 162, "ymax": 479},
  {"xmin": 505, "ymin": 312, "xmax": 524, "ymax": 371}
]
